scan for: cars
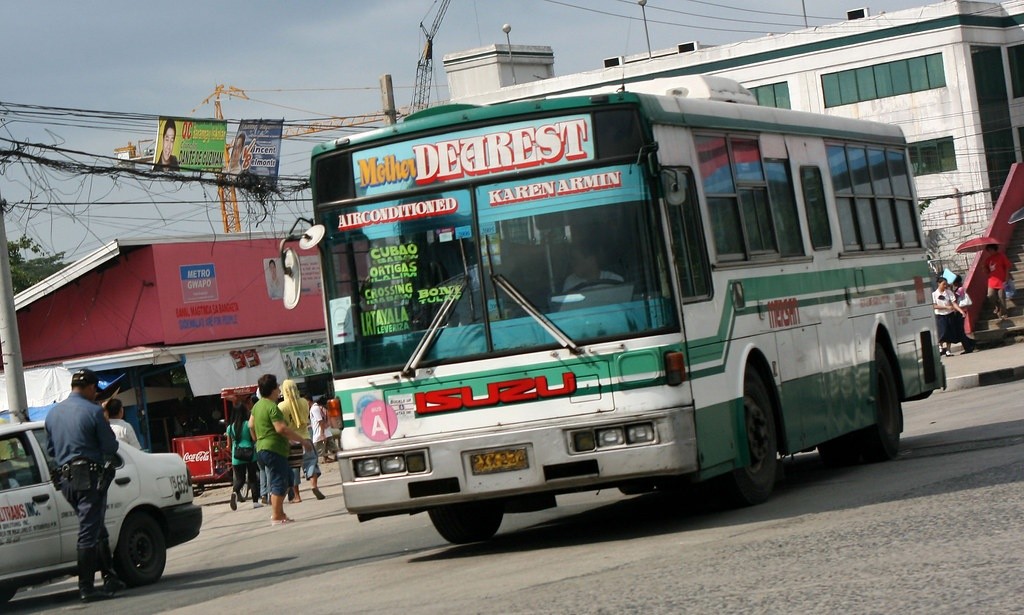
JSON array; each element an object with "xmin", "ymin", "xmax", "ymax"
[{"xmin": 0, "ymin": 421, "xmax": 203, "ymax": 605}]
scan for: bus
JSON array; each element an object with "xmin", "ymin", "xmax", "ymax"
[{"xmin": 278, "ymin": 76, "xmax": 948, "ymax": 546}]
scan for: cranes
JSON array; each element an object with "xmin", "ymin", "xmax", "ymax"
[{"xmin": 113, "ymin": 82, "xmax": 451, "ymax": 233}]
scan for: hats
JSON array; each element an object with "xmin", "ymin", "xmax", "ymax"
[{"xmin": 71, "ymin": 369, "xmax": 103, "ymax": 392}]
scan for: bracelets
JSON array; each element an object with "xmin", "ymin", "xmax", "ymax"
[{"xmin": 321, "ymin": 433, "xmax": 324, "ymax": 436}]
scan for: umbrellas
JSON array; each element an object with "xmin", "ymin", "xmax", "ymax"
[{"xmin": 956, "ymin": 235, "xmax": 1000, "ymax": 268}]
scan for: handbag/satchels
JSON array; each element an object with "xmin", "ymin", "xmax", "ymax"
[
  {"xmin": 288, "ymin": 443, "xmax": 304, "ymax": 468},
  {"xmin": 234, "ymin": 447, "xmax": 253, "ymax": 461},
  {"xmin": 957, "ymin": 293, "xmax": 972, "ymax": 307},
  {"xmin": 1002, "ymin": 279, "xmax": 1017, "ymax": 299}
]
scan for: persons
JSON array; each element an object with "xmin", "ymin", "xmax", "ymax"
[
  {"xmin": 985, "ymin": 244, "xmax": 1014, "ymax": 319},
  {"xmin": 153, "ymin": 120, "xmax": 180, "ymax": 172},
  {"xmin": 547, "ymin": 239, "xmax": 626, "ymax": 311},
  {"xmin": 304, "ymin": 393, "xmax": 338, "ymax": 463},
  {"xmin": 106, "ymin": 399, "xmax": 141, "ymax": 450},
  {"xmin": 248, "ymin": 374, "xmax": 310, "ymax": 524},
  {"xmin": 285, "ymin": 350, "xmax": 329, "ymax": 377},
  {"xmin": 932, "ymin": 274, "xmax": 978, "ymax": 356},
  {"xmin": 45, "ymin": 369, "xmax": 125, "ymax": 602},
  {"xmin": 226, "ymin": 396, "xmax": 272, "ymax": 511},
  {"xmin": 277, "ymin": 379, "xmax": 325, "ymax": 503},
  {"xmin": 11, "ymin": 442, "xmax": 18, "ymax": 458},
  {"xmin": 269, "ymin": 260, "xmax": 284, "ymax": 297},
  {"xmin": 229, "ymin": 133, "xmax": 246, "ymax": 180}
]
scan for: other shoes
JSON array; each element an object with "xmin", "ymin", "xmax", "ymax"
[
  {"xmin": 230, "ymin": 492, "xmax": 237, "ymax": 510},
  {"xmin": 946, "ymin": 350, "xmax": 954, "ymax": 356},
  {"xmin": 253, "ymin": 502, "xmax": 264, "ymax": 508},
  {"xmin": 102, "ymin": 572, "xmax": 126, "ymax": 590},
  {"xmin": 81, "ymin": 588, "xmax": 114, "ymax": 603}
]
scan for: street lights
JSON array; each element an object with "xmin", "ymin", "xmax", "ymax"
[
  {"xmin": 638, "ymin": 0, "xmax": 652, "ymax": 57},
  {"xmin": 503, "ymin": 24, "xmax": 517, "ymax": 84}
]
